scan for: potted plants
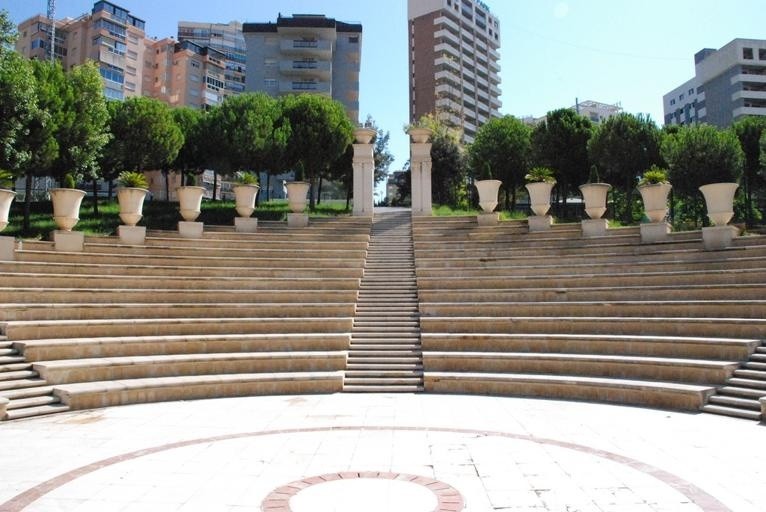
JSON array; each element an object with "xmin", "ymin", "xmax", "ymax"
[
  {"xmin": 232, "ymin": 169, "xmax": 261, "ymax": 216},
  {"xmin": 403, "ymin": 111, "xmax": 437, "ymax": 143},
  {"xmin": 474, "ymin": 161, "xmax": 502, "ymax": 213},
  {"xmin": 579, "ymin": 164, "xmax": 739, "ymax": 226},
  {"xmin": 0, "ymin": 168, "xmax": 17, "ymax": 234},
  {"xmin": 117, "ymin": 171, "xmax": 150, "ymax": 225},
  {"xmin": 48, "ymin": 173, "xmax": 87, "ymax": 231},
  {"xmin": 176, "ymin": 172, "xmax": 207, "ymax": 221},
  {"xmin": 525, "ymin": 165, "xmax": 557, "ymax": 215},
  {"xmin": 284, "ymin": 159, "xmax": 312, "ymax": 212}
]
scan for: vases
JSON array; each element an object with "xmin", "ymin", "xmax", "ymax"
[{"xmin": 351, "ymin": 128, "xmax": 377, "ymax": 143}]
